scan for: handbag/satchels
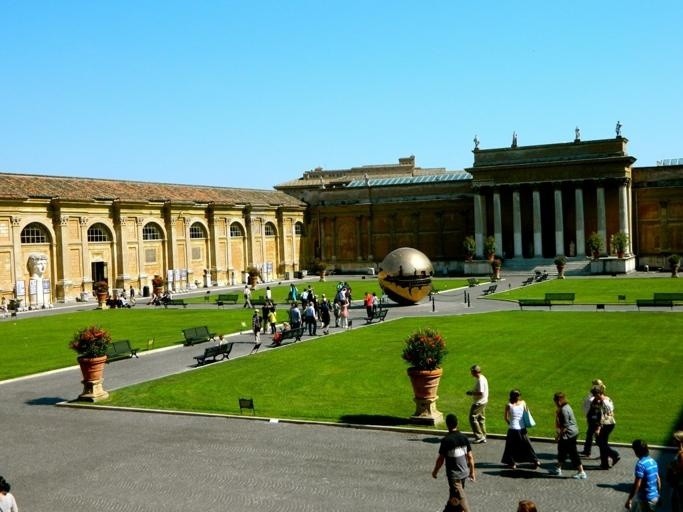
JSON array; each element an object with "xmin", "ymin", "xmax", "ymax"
[{"xmin": 523, "ymin": 410, "xmax": 536, "ymax": 426}]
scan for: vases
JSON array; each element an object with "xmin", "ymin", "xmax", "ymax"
[
  {"xmin": 76, "ymin": 355, "xmax": 107, "ymax": 382},
  {"xmin": 408, "ymin": 367, "xmax": 443, "ymax": 399},
  {"xmin": 402, "ymin": 325, "xmax": 449, "ymax": 371}
]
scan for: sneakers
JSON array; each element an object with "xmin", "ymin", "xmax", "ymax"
[
  {"xmin": 579, "ymin": 450, "xmax": 591, "ymax": 457},
  {"xmin": 596, "ymin": 456, "xmax": 620, "ymax": 470},
  {"xmin": 534, "ymin": 460, "xmax": 541, "ymax": 465},
  {"xmin": 473, "ymin": 437, "xmax": 486, "ymax": 443},
  {"xmin": 508, "ymin": 464, "xmax": 516, "ymax": 468},
  {"xmin": 549, "ymin": 467, "xmax": 561, "ymax": 474},
  {"xmin": 572, "ymin": 471, "xmax": 586, "ymax": 478}
]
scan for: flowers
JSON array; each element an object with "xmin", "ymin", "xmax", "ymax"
[{"xmin": 67, "ymin": 325, "xmax": 112, "ymax": 359}]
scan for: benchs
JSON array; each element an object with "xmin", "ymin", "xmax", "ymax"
[
  {"xmin": 96, "ymin": 291, "xmax": 389, "ymax": 365},
  {"xmin": 467, "ymin": 272, "xmax": 682, "ymax": 311}
]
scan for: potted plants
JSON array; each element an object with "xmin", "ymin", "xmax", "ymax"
[
  {"xmin": 151, "ymin": 276, "xmax": 164, "ymax": 296},
  {"xmin": 462, "ymin": 231, "xmax": 630, "ymax": 279},
  {"xmin": 247, "ymin": 267, "xmax": 259, "ymax": 290},
  {"xmin": 317, "ymin": 263, "xmax": 327, "ymax": 282}
]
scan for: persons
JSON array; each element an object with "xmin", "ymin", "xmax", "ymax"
[
  {"xmin": 541, "ymin": 270, "xmax": 547, "ymax": 277},
  {"xmin": 1, "ymin": 297, "xmax": 8, "ymax": 314},
  {"xmin": 431, "ymin": 413, "xmax": 476, "ymax": 512},
  {"xmin": 26, "ymin": 254, "xmax": 47, "ymax": 307},
  {"xmin": 218, "ymin": 335, "xmax": 228, "ymax": 346},
  {"xmin": 576, "ymin": 379, "xmax": 606, "ymax": 457},
  {"xmin": 149, "ymin": 291, "xmax": 173, "ymax": 306},
  {"xmin": 0, "ymin": 476, "xmax": 18, "ymax": 512},
  {"xmin": 465, "ymin": 364, "xmax": 489, "ymax": 443},
  {"xmin": 119, "ymin": 286, "xmax": 136, "ymax": 307},
  {"xmin": 242, "ymin": 280, "xmax": 381, "ymax": 354},
  {"xmin": 590, "ymin": 385, "xmax": 621, "ymax": 470},
  {"xmin": 501, "ymin": 388, "xmax": 541, "ymax": 470},
  {"xmin": 673, "ymin": 431, "xmax": 682, "ymax": 511},
  {"xmin": 548, "ymin": 393, "xmax": 587, "ymax": 480},
  {"xmin": 625, "ymin": 439, "xmax": 661, "ymax": 512},
  {"xmin": 516, "ymin": 501, "xmax": 537, "ymax": 512}
]
